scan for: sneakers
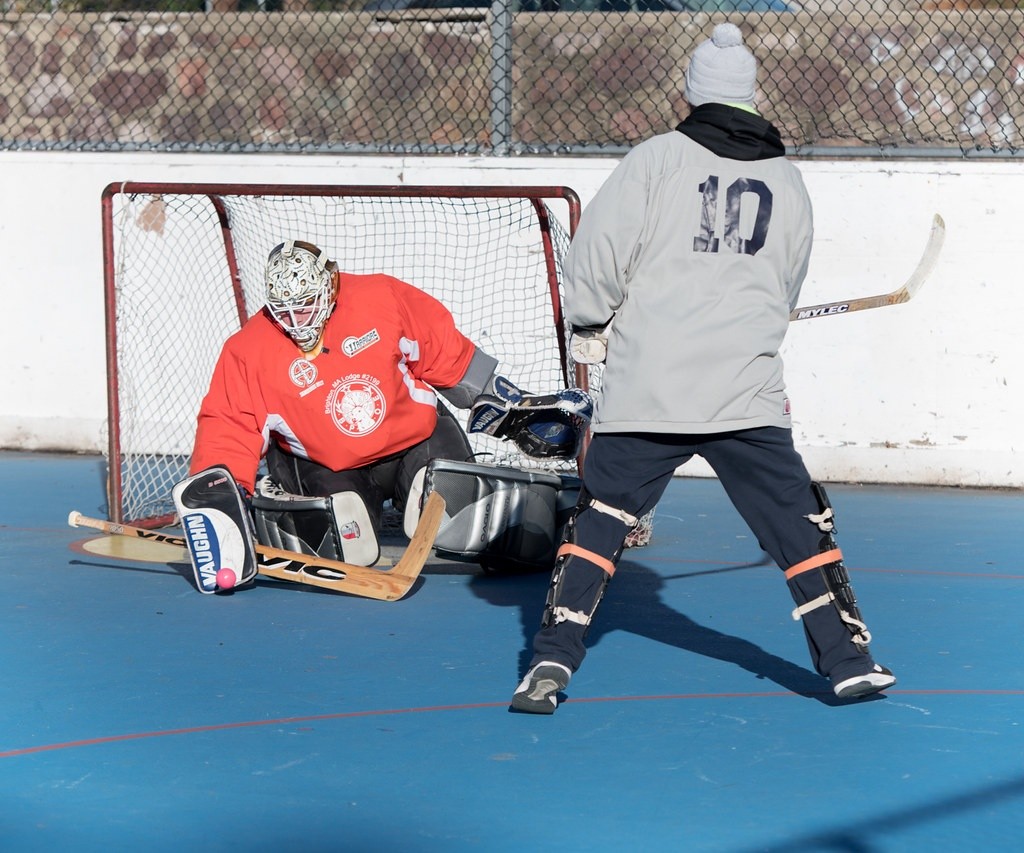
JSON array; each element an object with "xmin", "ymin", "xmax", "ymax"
[
  {"xmin": 834, "ymin": 664, "xmax": 895, "ymax": 700},
  {"xmin": 513, "ymin": 662, "xmax": 571, "ymax": 713}
]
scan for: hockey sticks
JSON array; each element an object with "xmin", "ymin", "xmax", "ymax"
[
  {"xmin": 68, "ymin": 489, "xmax": 447, "ymax": 602},
  {"xmin": 581, "ymin": 210, "xmax": 947, "ymax": 364}
]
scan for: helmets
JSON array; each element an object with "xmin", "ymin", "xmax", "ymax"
[{"xmin": 265, "ymin": 239, "xmax": 340, "ymax": 352}]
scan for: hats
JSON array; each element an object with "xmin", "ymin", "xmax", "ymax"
[{"xmin": 685, "ymin": 24, "xmax": 757, "ymax": 107}]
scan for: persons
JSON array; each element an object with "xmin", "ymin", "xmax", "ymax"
[
  {"xmin": 509, "ymin": 22, "xmax": 898, "ymax": 714},
  {"xmin": 170, "ymin": 241, "xmax": 595, "ymax": 596}
]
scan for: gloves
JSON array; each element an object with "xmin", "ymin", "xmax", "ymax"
[{"xmin": 570, "ymin": 311, "xmax": 614, "ymax": 364}]
